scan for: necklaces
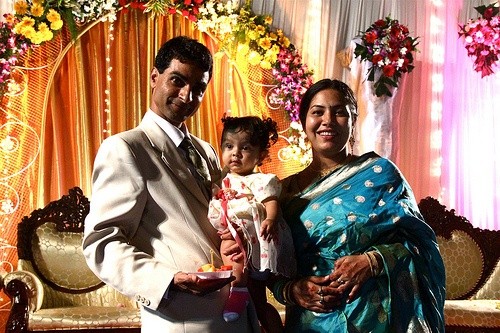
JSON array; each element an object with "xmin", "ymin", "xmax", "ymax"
[{"xmin": 309, "ymin": 153, "xmax": 352, "ymax": 182}]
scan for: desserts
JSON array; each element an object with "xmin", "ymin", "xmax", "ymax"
[{"xmin": 196, "ymin": 263, "xmax": 233, "ymax": 272}]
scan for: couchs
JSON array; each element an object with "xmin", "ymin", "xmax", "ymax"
[{"xmin": 2, "ymin": 186, "xmax": 500, "ymax": 333}]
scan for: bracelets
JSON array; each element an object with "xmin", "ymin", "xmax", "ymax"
[{"xmin": 363, "ymin": 252, "xmax": 379, "ymax": 277}]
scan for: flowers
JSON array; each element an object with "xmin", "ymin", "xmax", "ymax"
[
  {"xmin": 354, "ymin": 16, "xmax": 420, "ymax": 98},
  {"xmin": 0, "ymin": 0, "xmax": 315, "ymax": 151},
  {"xmin": 457, "ymin": 2, "xmax": 500, "ymax": 79}
]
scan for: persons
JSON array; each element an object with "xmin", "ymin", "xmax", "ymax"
[
  {"xmin": 266, "ymin": 78, "xmax": 446, "ymax": 333},
  {"xmin": 82, "ymin": 36, "xmax": 293, "ymax": 333},
  {"xmin": 208, "ymin": 112, "xmax": 282, "ymax": 323}
]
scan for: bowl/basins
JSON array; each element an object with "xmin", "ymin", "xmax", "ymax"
[{"xmin": 184, "ymin": 270, "xmax": 232, "ymax": 287}]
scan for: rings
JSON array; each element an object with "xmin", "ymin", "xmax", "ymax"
[
  {"xmin": 319, "ymin": 294, "xmax": 326, "ymax": 304},
  {"xmin": 317, "ymin": 286, "xmax": 325, "ymax": 295},
  {"xmin": 337, "ymin": 278, "xmax": 345, "ymax": 285}
]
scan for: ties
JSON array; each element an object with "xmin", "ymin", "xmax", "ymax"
[{"xmin": 178, "ymin": 135, "xmax": 213, "ymax": 201}]
